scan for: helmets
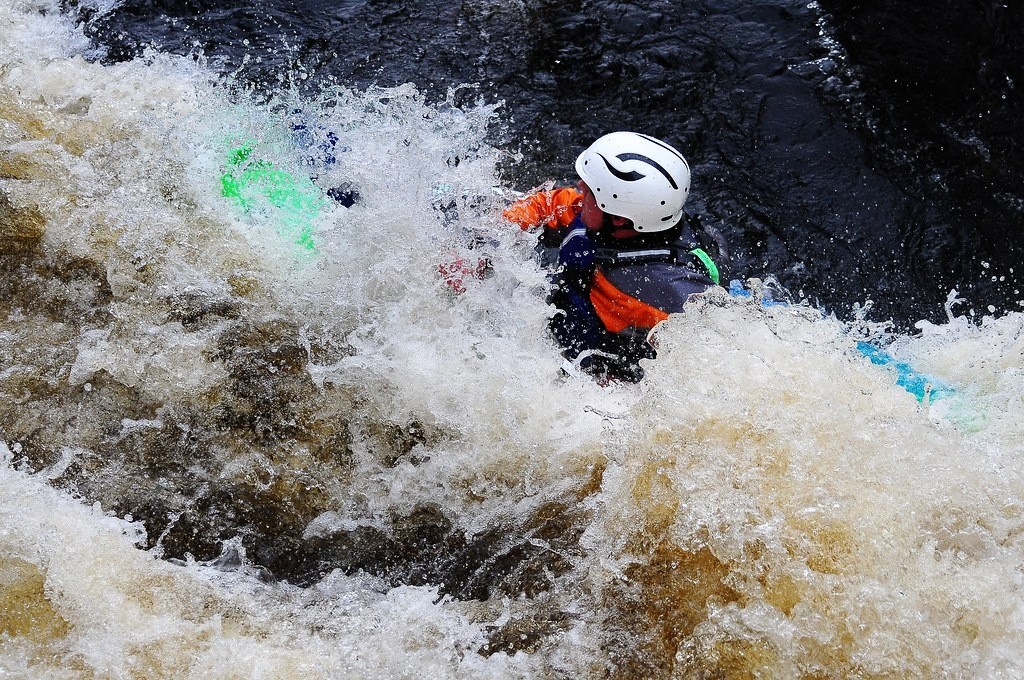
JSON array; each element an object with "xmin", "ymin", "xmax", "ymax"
[{"xmin": 574, "ymin": 131, "xmax": 691, "ymax": 232}]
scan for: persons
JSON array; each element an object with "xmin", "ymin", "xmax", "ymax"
[{"xmin": 441, "ymin": 131, "xmax": 726, "ymax": 388}]
